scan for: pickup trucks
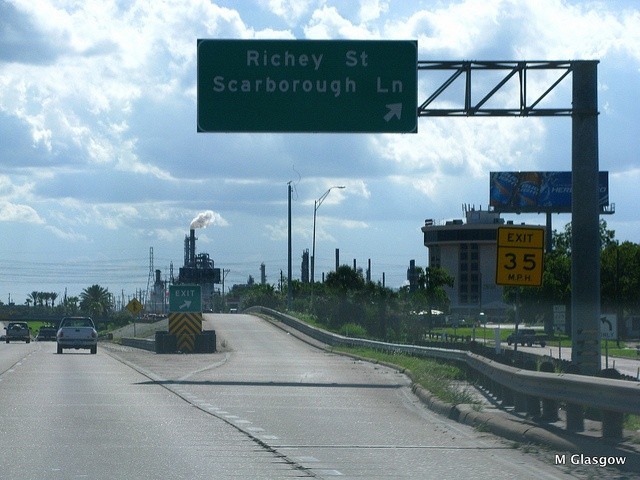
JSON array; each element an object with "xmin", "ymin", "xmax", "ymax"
[
  {"xmin": 507, "ymin": 329, "xmax": 549, "ymax": 347},
  {"xmin": 4, "ymin": 322, "xmax": 32, "ymax": 343},
  {"xmin": 56, "ymin": 317, "xmax": 98, "ymax": 354}
]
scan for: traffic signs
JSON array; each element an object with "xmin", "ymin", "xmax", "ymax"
[
  {"xmin": 496, "ymin": 226, "xmax": 544, "ymax": 286},
  {"xmin": 197, "ymin": 39, "xmax": 418, "ymax": 133}
]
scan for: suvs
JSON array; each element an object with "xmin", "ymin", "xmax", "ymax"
[{"xmin": 36, "ymin": 325, "xmax": 57, "ymax": 342}]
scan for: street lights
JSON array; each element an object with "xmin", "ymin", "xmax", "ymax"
[{"xmin": 311, "ymin": 185, "xmax": 346, "ymax": 314}]
